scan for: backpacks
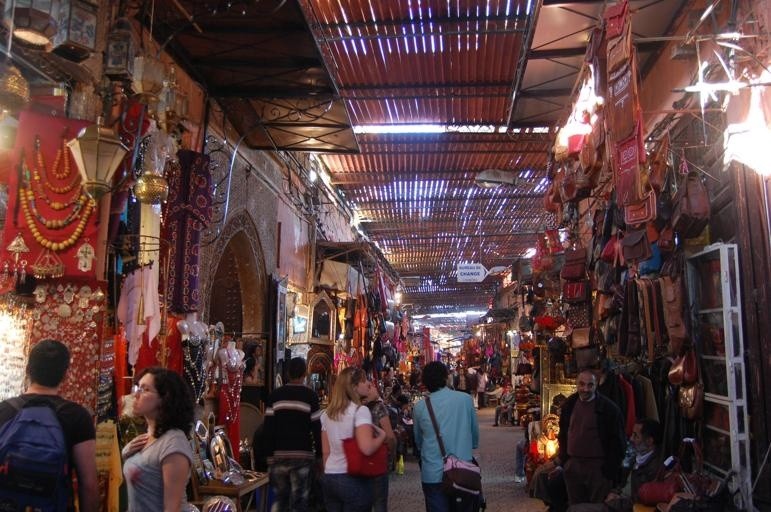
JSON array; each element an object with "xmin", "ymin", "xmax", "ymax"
[{"xmin": 1, "ymin": 397, "xmax": 78, "ymax": 512}]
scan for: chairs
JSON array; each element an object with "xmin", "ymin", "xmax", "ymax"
[
  {"xmin": 192, "ymin": 412, "xmax": 270, "ymax": 512},
  {"xmin": 239, "ymin": 401, "xmax": 265, "ymax": 445}
]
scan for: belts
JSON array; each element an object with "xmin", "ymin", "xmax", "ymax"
[{"xmin": 615, "ymin": 275, "xmax": 662, "ymax": 364}]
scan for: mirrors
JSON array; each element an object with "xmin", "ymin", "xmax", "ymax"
[{"xmin": 310, "ymin": 359, "xmax": 329, "ymax": 404}]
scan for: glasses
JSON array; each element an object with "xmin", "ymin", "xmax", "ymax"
[{"xmin": 131, "ymin": 384, "xmax": 157, "ymax": 394}]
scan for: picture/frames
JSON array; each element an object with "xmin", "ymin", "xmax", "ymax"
[{"xmin": 543, "ymin": 384, "xmax": 578, "ymax": 417}]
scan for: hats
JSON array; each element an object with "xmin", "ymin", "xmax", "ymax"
[
  {"xmin": 383, "ymin": 345, "xmax": 401, "ymax": 371},
  {"xmin": 547, "ymin": 336, "xmax": 567, "ymax": 363},
  {"xmin": 532, "ymin": 277, "xmax": 545, "ymax": 297}
]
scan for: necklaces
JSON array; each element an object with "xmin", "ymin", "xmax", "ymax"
[{"xmin": 12, "ymin": 121, "xmax": 98, "ymax": 254}]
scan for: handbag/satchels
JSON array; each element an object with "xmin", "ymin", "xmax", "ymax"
[
  {"xmin": 678, "ymin": 384, "xmax": 705, "ymax": 420},
  {"xmin": 561, "ymin": 277, "xmax": 620, "ymax": 369},
  {"xmin": 501, "ymin": 405, "xmax": 509, "ymax": 414},
  {"xmin": 442, "ymin": 454, "xmax": 487, "ymax": 511},
  {"xmin": 643, "ymin": 135, "xmax": 669, "ymax": 196},
  {"xmin": 367, "ymin": 313, "xmax": 386, "ymax": 368},
  {"xmin": 545, "ymin": 136, "xmax": 593, "ymax": 212},
  {"xmin": 667, "ymin": 173, "xmax": 710, "ymax": 239},
  {"xmin": 544, "ymin": 209, "xmax": 558, "ymax": 231},
  {"xmin": 364, "ymin": 268, "xmax": 387, "ymax": 312},
  {"xmin": 623, "ymin": 191, "xmax": 680, "ymax": 280},
  {"xmin": 667, "ymin": 345, "xmax": 697, "ymax": 388},
  {"xmin": 513, "ymin": 315, "xmax": 535, "ymax": 375},
  {"xmin": 639, "ymin": 437, "xmax": 741, "ymax": 512},
  {"xmin": 340, "ymin": 406, "xmax": 388, "ymax": 483},
  {"xmin": 560, "ymin": 204, "xmax": 619, "ymax": 296}
]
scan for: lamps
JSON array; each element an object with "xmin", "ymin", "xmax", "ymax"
[
  {"xmin": 104, "ymin": 17, "xmax": 136, "ymax": 82},
  {"xmin": 66, "ymin": 113, "xmax": 130, "ymax": 208},
  {"xmin": 46, "ymin": 0, "xmax": 99, "ymax": 64}
]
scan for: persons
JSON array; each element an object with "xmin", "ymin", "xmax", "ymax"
[
  {"xmin": 177, "ymin": 313, "xmax": 211, "ymax": 407},
  {"xmin": 261, "ymin": 355, "xmax": 324, "ymax": 511},
  {"xmin": 412, "ymin": 360, "xmax": 488, "ymax": 511},
  {"xmin": 602, "ymin": 415, "xmax": 668, "ymax": 511},
  {"xmin": 319, "ymin": 365, "xmax": 388, "ymax": 511},
  {"xmin": 560, "ymin": 368, "xmax": 629, "ymax": 511},
  {"xmin": 214, "ymin": 337, "xmax": 246, "ymax": 460},
  {"xmin": 122, "ymin": 365, "xmax": 198, "ymax": 512},
  {"xmin": 1, "ymin": 338, "xmax": 101, "ymax": 511},
  {"xmin": 236, "ymin": 336, "xmax": 514, "ymax": 471},
  {"xmin": 360, "ymin": 380, "xmax": 397, "ymax": 511}
]
